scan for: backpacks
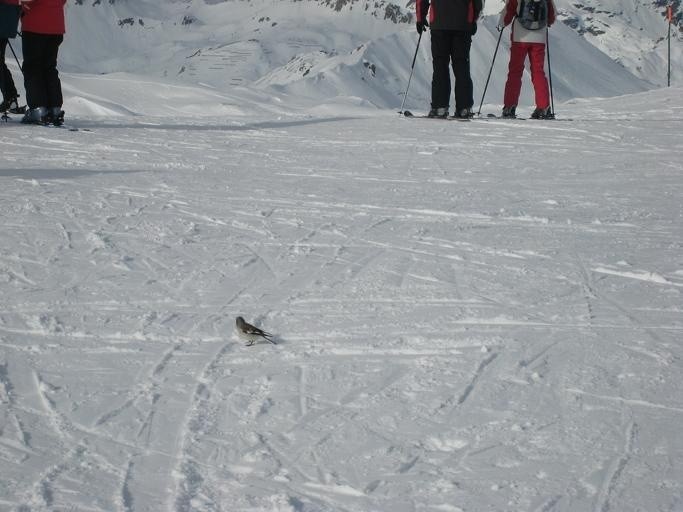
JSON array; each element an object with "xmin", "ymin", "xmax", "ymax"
[{"xmin": 519, "ymin": 0, "xmax": 547, "ymax": 30}]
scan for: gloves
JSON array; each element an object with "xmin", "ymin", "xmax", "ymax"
[
  {"xmin": 471, "ymin": 22, "xmax": 477, "ymax": 35},
  {"xmin": 415, "ymin": 20, "xmax": 429, "ymax": 34},
  {"xmin": 496, "ymin": 24, "xmax": 504, "ymax": 33}
]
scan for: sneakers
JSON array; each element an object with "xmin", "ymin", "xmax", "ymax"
[
  {"xmin": 47, "ymin": 105, "xmax": 64, "ymax": 126},
  {"xmin": 454, "ymin": 108, "xmax": 473, "ymax": 117},
  {"xmin": 501, "ymin": 105, "xmax": 516, "ymax": 117},
  {"xmin": 23, "ymin": 106, "xmax": 48, "ymax": 125},
  {"xmin": 531, "ymin": 105, "xmax": 552, "ymax": 118},
  {"xmin": 427, "ymin": 105, "xmax": 451, "ymax": 118}
]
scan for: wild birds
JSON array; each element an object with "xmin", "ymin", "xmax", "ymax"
[{"xmin": 236, "ymin": 317, "xmax": 277, "ymax": 346}]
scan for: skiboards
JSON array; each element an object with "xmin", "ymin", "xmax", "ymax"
[
  {"xmin": 404, "ymin": 111, "xmax": 573, "ymax": 122},
  {"xmin": 44, "ymin": 124, "xmax": 90, "ymax": 131}
]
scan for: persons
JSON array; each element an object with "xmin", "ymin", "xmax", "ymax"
[
  {"xmin": 19, "ymin": 0, "xmax": 65, "ymax": 124},
  {"xmin": 0, "ymin": 2, "xmax": 20, "ymax": 111},
  {"xmin": 498, "ymin": 0, "xmax": 557, "ymax": 119},
  {"xmin": 415, "ymin": 1, "xmax": 484, "ymax": 118}
]
